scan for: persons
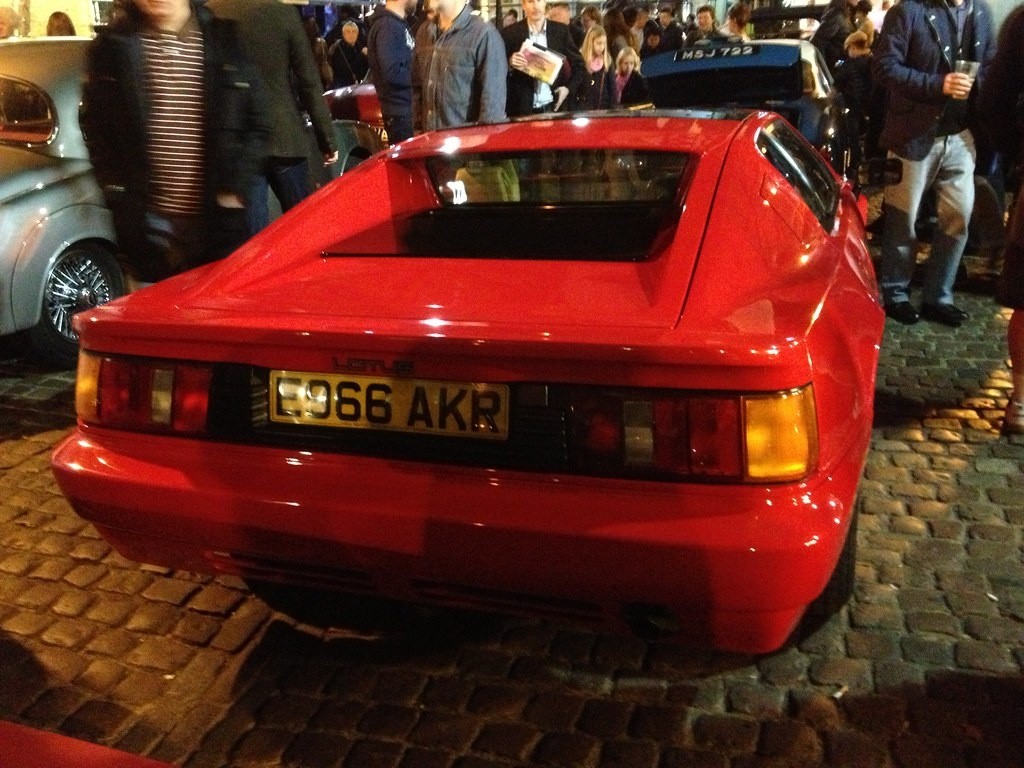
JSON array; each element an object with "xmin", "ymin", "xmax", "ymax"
[
  {"xmin": 368, "ymin": 0, "xmax": 417, "ymax": 141},
  {"xmin": 80, "ymin": 0, "xmax": 260, "ymax": 277},
  {"xmin": 501, "ymin": 0, "xmax": 586, "ymax": 117},
  {"xmin": 991, "ymin": 3, "xmax": 1024, "ymax": 443},
  {"xmin": 877, "ymin": 0, "xmax": 994, "ymax": 324},
  {"xmin": 306, "ymin": 17, "xmax": 333, "ymax": 91},
  {"xmin": 331, "ymin": 21, "xmax": 370, "ymax": 89},
  {"xmin": 503, "ymin": 9, "xmax": 519, "ymax": 28},
  {"xmin": 202, "ymin": 0, "xmax": 338, "ymax": 241},
  {"xmin": 411, "ymin": 0, "xmax": 508, "ymax": 135},
  {"xmin": 543, "ymin": 1, "xmax": 878, "ymax": 198}
]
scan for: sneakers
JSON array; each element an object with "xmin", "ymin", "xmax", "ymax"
[
  {"xmin": 884, "ymin": 298, "xmax": 920, "ymax": 324},
  {"xmin": 922, "ymin": 301, "xmax": 970, "ymax": 327}
]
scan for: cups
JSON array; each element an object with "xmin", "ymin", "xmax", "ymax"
[{"xmin": 951, "ymin": 59, "xmax": 980, "ymax": 99}]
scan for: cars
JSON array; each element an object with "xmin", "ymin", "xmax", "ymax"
[
  {"xmin": 589, "ymin": 38, "xmax": 837, "ymax": 147},
  {"xmin": 319, "ymin": 82, "xmax": 387, "ymax": 161},
  {"xmin": 49, "ymin": 108, "xmax": 888, "ymax": 657},
  {"xmin": 0, "ymin": 38, "xmax": 383, "ymax": 368}
]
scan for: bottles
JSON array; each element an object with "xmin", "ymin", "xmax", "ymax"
[{"xmin": 835, "ymin": 60, "xmax": 845, "ymax": 67}]
